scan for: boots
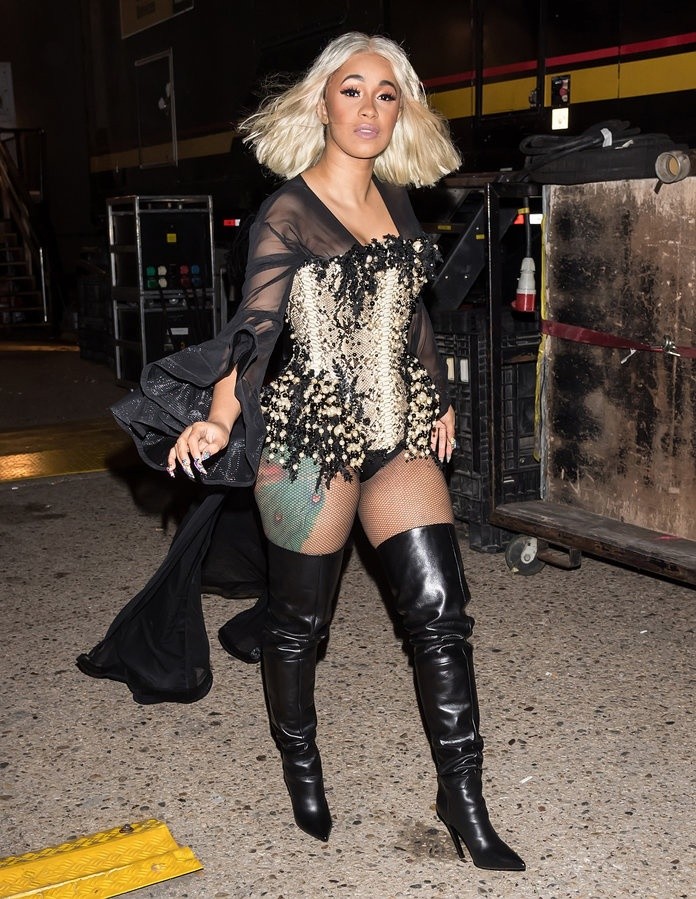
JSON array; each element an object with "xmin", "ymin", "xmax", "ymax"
[
  {"xmin": 370, "ymin": 524, "xmax": 527, "ymax": 871},
  {"xmin": 258, "ymin": 529, "xmax": 346, "ymax": 843}
]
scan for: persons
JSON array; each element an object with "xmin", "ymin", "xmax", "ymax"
[{"xmin": 74, "ymin": 31, "xmax": 528, "ymax": 871}]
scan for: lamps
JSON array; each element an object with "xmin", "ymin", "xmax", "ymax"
[{"xmin": 654, "ymin": 150, "xmax": 696, "ymax": 183}]
